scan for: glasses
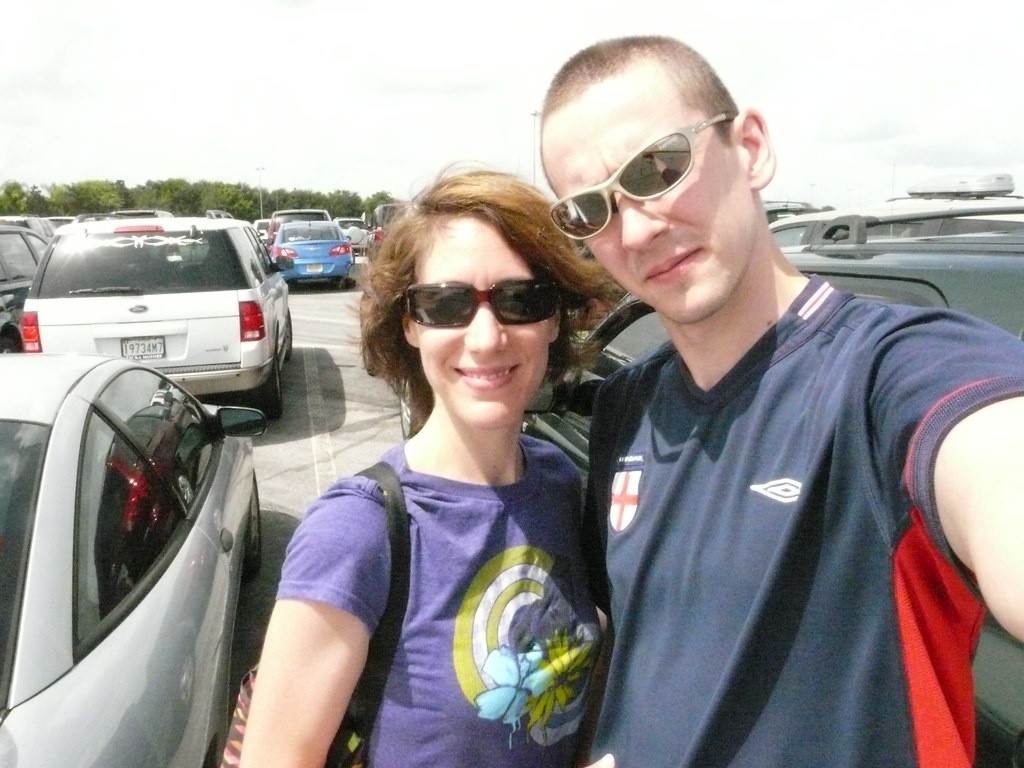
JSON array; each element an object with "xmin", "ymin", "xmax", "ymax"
[
  {"xmin": 548, "ymin": 112, "xmax": 737, "ymax": 240},
  {"xmin": 405, "ymin": 279, "xmax": 562, "ymax": 328}
]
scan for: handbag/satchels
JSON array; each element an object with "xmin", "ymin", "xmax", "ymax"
[{"xmin": 222, "ymin": 463, "xmax": 411, "ymax": 768}]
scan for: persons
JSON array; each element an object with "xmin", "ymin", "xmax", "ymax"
[
  {"xmin": 441, "ymin": 36, "xmax": 1022, "ymax": 768},
  {"xmin": 238, "ymin": 162, "xmax": 608, "ymax": 768}
]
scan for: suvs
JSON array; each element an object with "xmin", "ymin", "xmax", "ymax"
[
  {"xmin": 516, "ymin": 185, "xmax": 1024, "ymax": 489},
  {"xmin": 21, "ymin": 208, "xmax": 297, "ymax": 416}
]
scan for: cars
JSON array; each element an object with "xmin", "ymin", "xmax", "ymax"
[
  {"xmin": 40, "ymin": 199, "xmax": 408, "ymax": 269},
  {"xmin": 269, "ymin": 221, "xmax": 357, "ymax": 288},
  {"xmin": 2, "ymin": 349, "xmax": 265, "ymax": 768},
  {"xmin": 1, "ymin": 224, "xmax": 54, "ymax": 354}
]
